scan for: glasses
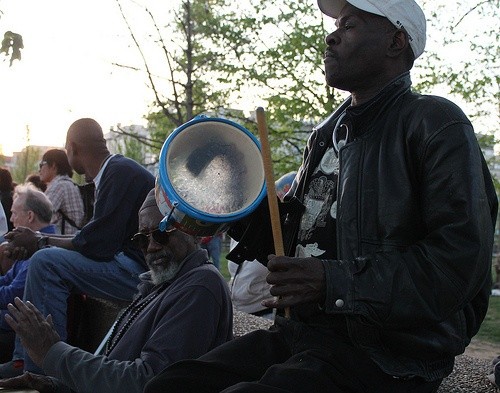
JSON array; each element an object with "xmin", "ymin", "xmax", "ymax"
[
  {"xmin": 39, "ymin": 163, "xmax": 46, "ymax": 168},
  {"xmin": 131, "ymin": 227, "xmax": 176, "ymax": 249}
]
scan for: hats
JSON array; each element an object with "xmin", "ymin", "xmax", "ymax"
[
  {"xmin": 318, "ymin": 0, "xmax": 426, "ymax": 59},
  {"xmin": 138, "ymin": 187, "xmax": 156, "ymax": 213}
]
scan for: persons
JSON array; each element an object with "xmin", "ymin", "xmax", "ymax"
[
  {"xmin": 144, "ymin": 0, "xmax": 499, "ymax": 392},
  {"xmin": 0, "ymin": 118, "xmax": 297, "ymax": 380},
  {"xmin": 0, "ymin": 187, "xmax": 233, "ymax": 393}
]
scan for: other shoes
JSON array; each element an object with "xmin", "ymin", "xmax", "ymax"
[{"xmin": 0, "ymin": 360, "xmax": 25, "ymax": 382}]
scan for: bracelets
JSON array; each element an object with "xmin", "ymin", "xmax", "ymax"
[{"xmin": 36, "ymin": 237, "xmax": 48, "ymax": 250}]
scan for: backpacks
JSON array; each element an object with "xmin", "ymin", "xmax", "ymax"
[{"xmin": 57, "ymin": 179, "xmax": 95, "ymax": 230}]
